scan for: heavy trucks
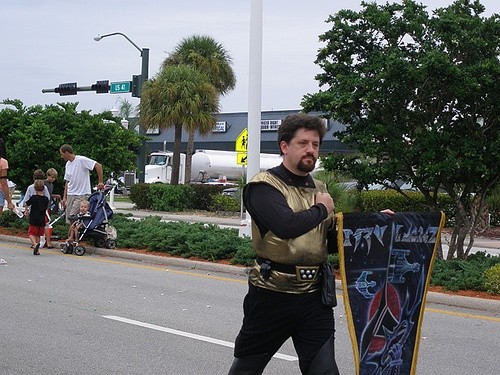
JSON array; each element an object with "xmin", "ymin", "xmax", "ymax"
[{"xmin": 144, "ymin": 149, "xmax": 328, "ymax": 184}]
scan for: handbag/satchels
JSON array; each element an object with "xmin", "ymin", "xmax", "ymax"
[{"xmin": 321, "ymin": 262, "xmax": 338, "ymax": 307}]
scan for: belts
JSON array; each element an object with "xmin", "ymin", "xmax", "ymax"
[{"xmin": 255, "ymin": 255, "xmax": 324, "ymax": 275}]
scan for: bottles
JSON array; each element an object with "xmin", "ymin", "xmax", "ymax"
[{"xmin": 12, "ymin": 207, "xmax": 24, "ymax": 219}]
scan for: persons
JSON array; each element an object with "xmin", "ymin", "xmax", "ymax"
[
  {"xmin": 60, "ymin": 144, "xmax": 104, "ymax": 252},
  {"xmin": 23, "ymin": 168, "xmax": 58, "ymax": 248},
  {"xmin": 24, "ymin": 180, "xmax": 49, "ymax": 255},
  {"xmin": 228, "ymin": 113, "xmax": 395, "ymax": 375},
  {"xmin": 66, "ymin": 200, "xmax": 91, "ymax": 245},
  {"xmin": 0, "ymin": 138, "xmax": 15, "ymax": 263}
]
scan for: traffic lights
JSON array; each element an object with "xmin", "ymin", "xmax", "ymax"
[
  {"xmin": 54, "ymin": 82, "xmax": 77, "ymax": 96},
  {"xmin": 94, "ymin": 79, "xmax": 110, "ymax": 93}
]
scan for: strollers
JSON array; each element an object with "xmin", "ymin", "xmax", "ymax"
[{"xmin": 59, "ymin": 184, "xmax": 116, "ymax": 256}]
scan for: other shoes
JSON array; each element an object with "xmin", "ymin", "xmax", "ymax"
[{"xmin": 34, "ymin": 243, "xmax": 40, "ymax": 255}]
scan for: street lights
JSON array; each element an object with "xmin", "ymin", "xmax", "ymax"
[{"xmin": 94, "ymin": 32, "xmax": 150, "ymax": 184}]
